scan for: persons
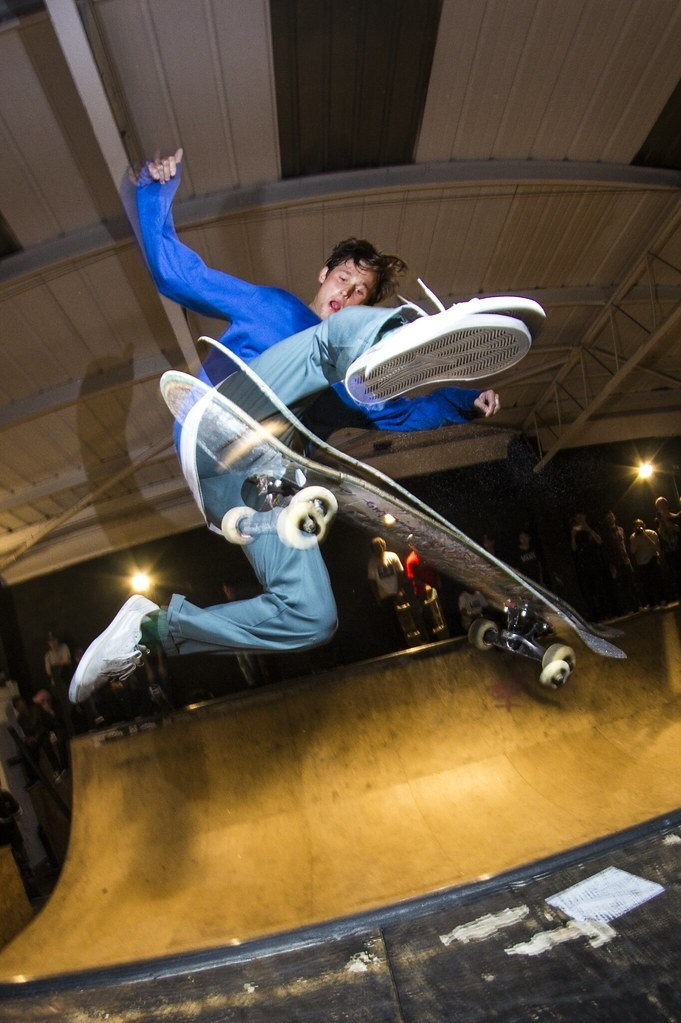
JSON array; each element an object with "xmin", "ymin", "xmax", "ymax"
[
  {"xmin": 478, "ymin": 532, "xmax": 496, "ymax": 557},
  {"xmin": 571, "ymin": 497, "xmax": 681, "ymax": 619},
  {"xmin": 140, "ymin": 644, "xmax": 168, "ymax": 684},
  {"xmin": 367, "ymin": 537, "xmax": 408, "ymax": 652},
  {"xmin": 221, "ymin": 581, "xmax": 271, "ymax": 685},
  {"xmin": 405, "ymin": 534, "xmax": 449, "ymax": 642},
  {"xmin": 506, "ymin": 531, "xmax": 546, "ymax": 588},
  {"xmin": 44, "ymin": 632, "xmax": 72, "ymax": 686},
  {"xmin": 68, "ymin": 148, "xmax": 549, "ymax": 704},
  {"xmin": 12, "ymin": 689, "xmax": 82, "ymax": 767}
]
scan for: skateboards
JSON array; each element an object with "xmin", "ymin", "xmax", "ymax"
[{"xmin": 154, "ymin": 368, "xmax": 630, "ymax": 691}]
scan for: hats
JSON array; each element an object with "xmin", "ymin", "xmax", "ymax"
[{"xmin": 32, "ymin": 689, "xmax": 50, "ymax": 704}]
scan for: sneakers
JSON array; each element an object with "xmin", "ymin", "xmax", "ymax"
[
  {"xmin": 68, "ymin": 594, "xmax": 160, "ymax": 705},
  {"xmin": 345, "ymin": 280, "xmax": 547, "ymax": 404}
]
